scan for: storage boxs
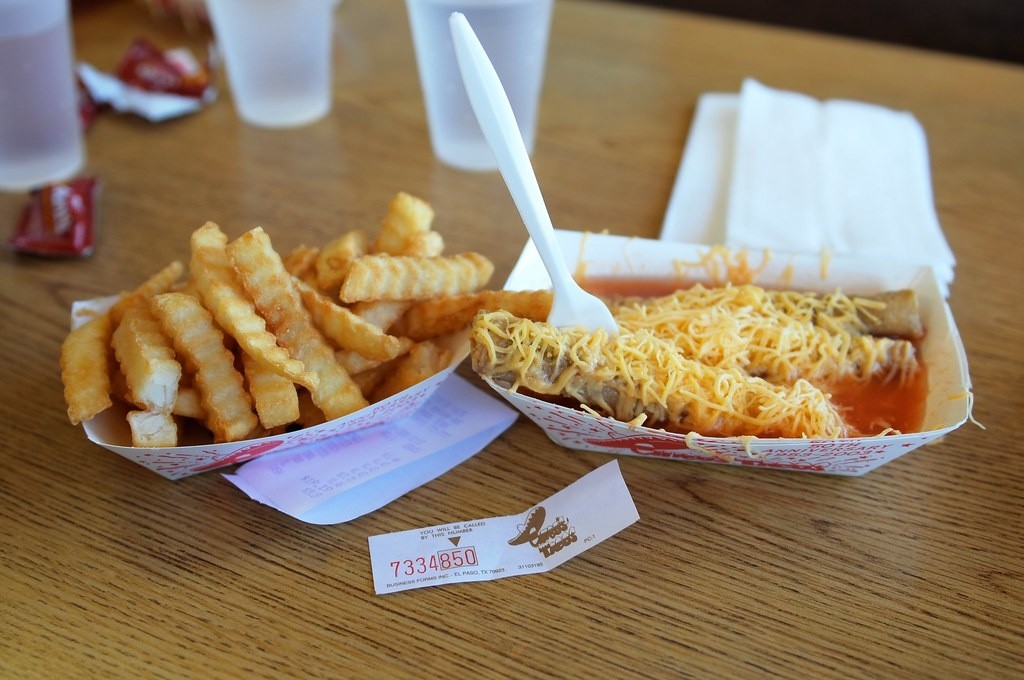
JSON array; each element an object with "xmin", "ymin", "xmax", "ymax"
[
  {"xmin": 69, "ymin": 295, "xmax": 474, "ymax": 483},
  {"xmin": 479, "ymin": 228, "xmax": 971, "ymax": 477}
]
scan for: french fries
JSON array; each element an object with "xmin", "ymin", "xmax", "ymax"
[{"xmin": 60, "ymin": 193, "xmax": 555, "ymax": 448}]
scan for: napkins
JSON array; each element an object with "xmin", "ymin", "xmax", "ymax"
[{"xmin": 659, "ymin": 77, "xmax": 956, "ymax": 300}]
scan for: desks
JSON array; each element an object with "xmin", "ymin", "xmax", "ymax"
[{"xmin": 0, "ymin": 0, "xmax": 1023, "ymax": 680}]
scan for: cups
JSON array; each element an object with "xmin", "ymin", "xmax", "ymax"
[
  {"xmin": 404, "ymin": 0, "xmax": 554, "ymax": 171},
  {"xmin": 1, "ymin": 0, "xmax": 84, "ymax": 191},
  {"xmin": 205, "ymin": 1, "xmax": 333, "ymax": 130}
]
